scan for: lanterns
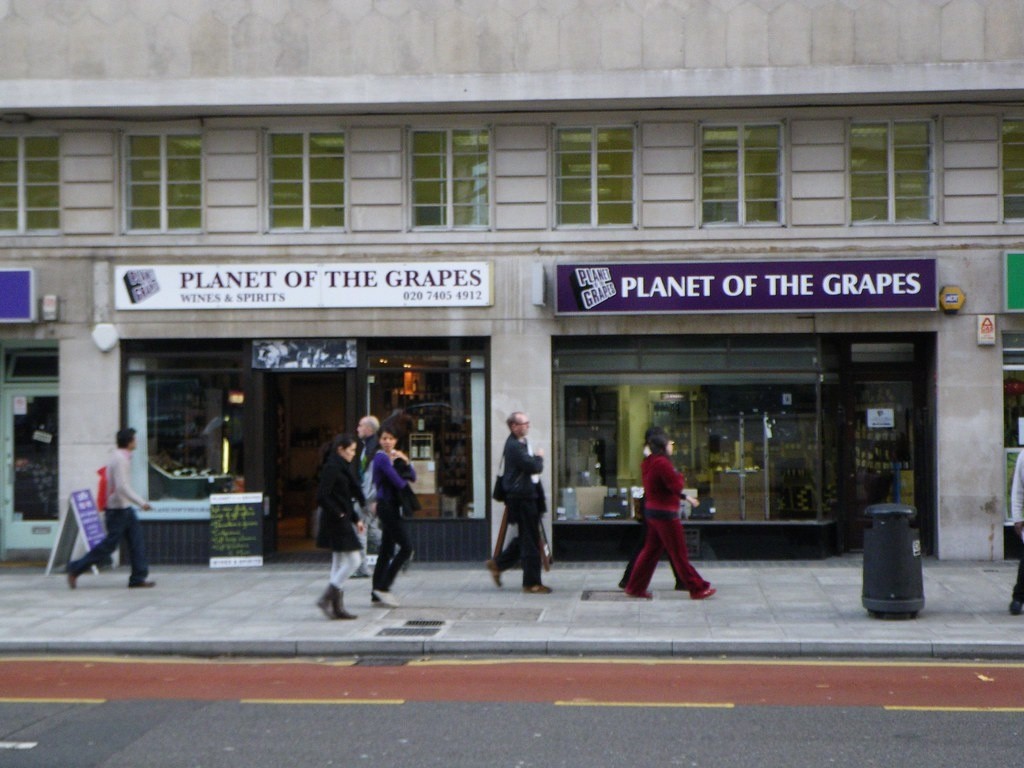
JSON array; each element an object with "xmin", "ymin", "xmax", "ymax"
[{"xmin": 1004, "ymin": 377, "xmax": 1024, "ymax": 406}]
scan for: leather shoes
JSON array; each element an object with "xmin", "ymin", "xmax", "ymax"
[
  {"xmin": 1010, "ymin": 600, "xmax": 1022, "ymax": 614},
  {"xmin": 318, "ymin": 584, "xmax": 357, "ymax": 619}
]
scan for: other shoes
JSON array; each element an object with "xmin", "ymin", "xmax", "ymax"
[
  {"xmin": 487, "ymin": 560, "xmax": 502, "ymax": 587},
  {"xmin": 128, "ymin": 580, "xmax": 155, "ymax": 588},
  {"xmin": 619, "ymin": 582, "xmax": 652, "ymax": 598},
  {"xmin": 370, "ymin": 589, "xmax": 400, "ymax": 608},
  {"xmin": 66, "ymin": 562, "xmax": 77, "ymax": 588},
  {"xmin": 523, "ymin": 584, "xmax": 552, "ymax": 594},
  {"xmin": 676, "ymin": 586, "xmax": 716, "ymax": 599}
]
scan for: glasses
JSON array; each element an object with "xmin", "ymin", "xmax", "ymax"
[
  {"xmin": 516, "ymin": 421, "xmax": 528, "ymax": 425},
  {"xmin": 668, "ymin": 442, "xmax": 675, "ymax": 446}
]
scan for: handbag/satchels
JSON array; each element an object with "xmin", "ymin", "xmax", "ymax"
[
  {"xmin": 97, "ymin": 466, "xmax": 107, "ymax": 511},
  {"xmin": 493, "ymin": 476, "xmax": 504, "ymax": 501}
]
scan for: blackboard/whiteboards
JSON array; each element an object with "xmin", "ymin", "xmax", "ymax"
[{"xmin": 208, "ymin": 491, "xmax": 263, "ymax": 569}]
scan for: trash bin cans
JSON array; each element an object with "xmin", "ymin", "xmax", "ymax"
[{"xmin": 860, "ymin": 502, "xmax": 926, "ymax": 620}]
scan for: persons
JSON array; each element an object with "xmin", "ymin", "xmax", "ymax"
[
  {"xmin": 316, "ymin": 416, "xmax": 413, "ymax": 620},
  {"xmin": 1008, "ymin": 450, "xmax": 1024, "ymax": 616},
  {"xmin": 486, "ymin": 412, "xmax": 551, "ymax": 592},
  {"xmin": 619, "ymin": 426, "xmax": 715, "ymax": 600},
  {"xmin": 66, "ymin": 427, "xmax": 156, "ymax": 588}
]
